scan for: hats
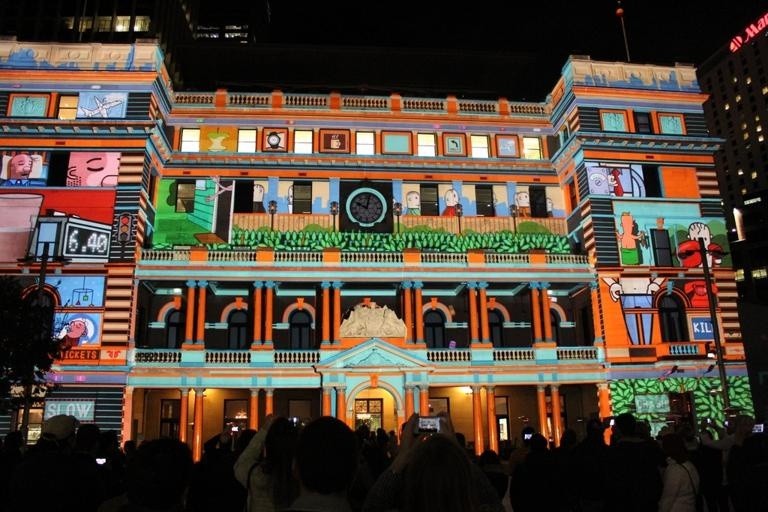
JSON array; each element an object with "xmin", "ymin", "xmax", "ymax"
[{"xmin": 41, "ymin": 416, "xmax": 76, "ymax": 443}]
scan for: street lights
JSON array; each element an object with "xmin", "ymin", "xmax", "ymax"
[
  {"xmin": 16, "ymin": 242, "xmax": 74, "ymax": 449},
  {"xmin": 672, "ymin": 238, "xmax": 744, "ymax": 420}
]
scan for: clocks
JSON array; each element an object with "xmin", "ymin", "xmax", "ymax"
[{"xmin": 344, "ymin": 186, "xmax": 389, "ymax": 229}]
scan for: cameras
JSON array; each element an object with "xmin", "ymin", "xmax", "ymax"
[
  {"xmin": 704, "ymin": 417, "xmax": 765, "ymax": 437},
  {"xmin": 523, "ymin": 433, "xmax": 534, "ymax": 441},
  {"xmin": 231, "ymin": 425, "xmax": 240, "ymax": 433},
  {"xmin": 413, "ymin": 415, "xmax": 449, "ymax": 436},
  {"xmin": 607, "ymin": 416, "xmax": 617, "ymax": 426},
  {"xmin": 289, "ymin": 416, "xmax": 300, "ymax": 426}
]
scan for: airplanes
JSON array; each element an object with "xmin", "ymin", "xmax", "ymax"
[{"xmin": 80, "ymin": 96, "xmax": 122, "ymax": 117}]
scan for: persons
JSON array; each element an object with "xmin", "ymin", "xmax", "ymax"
[{"xmin": 0, "ymin": 409, "xmax": 768, "ymax": 512}]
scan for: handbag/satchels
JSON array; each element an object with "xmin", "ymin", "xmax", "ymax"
[{"xmin": 694, "ymin": 493, "xmax": 704, "ymax": 512}]
